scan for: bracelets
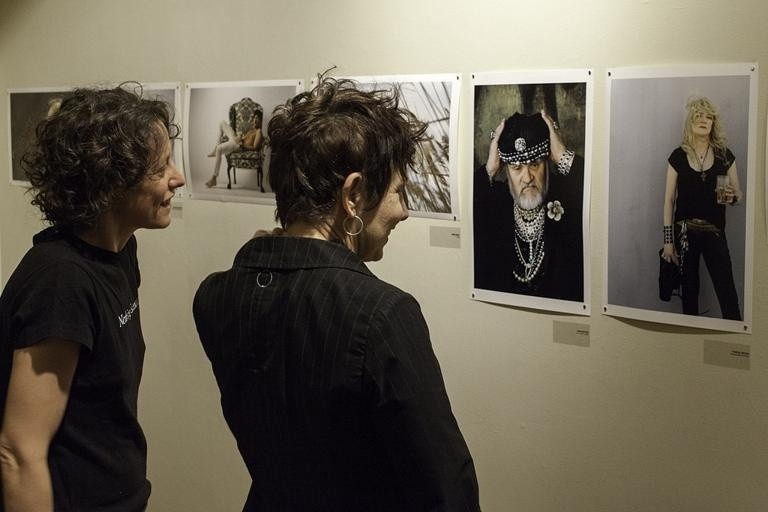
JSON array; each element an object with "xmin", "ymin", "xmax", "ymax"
[
  {"xmin": 556, "ymin": 148, "xmax": 575, "ymax": 176},
  {"xmin": 664, "ymin": 224, "xmax": 674, "ymax": 245},
  {"xmin": 483, "ymin": 165, "xmax": 495, "ymax": 188}
]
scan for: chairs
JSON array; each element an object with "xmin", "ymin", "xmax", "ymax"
[{"xmin": 221, "ymin": 97, "xmax": 269, "ymax": 192}]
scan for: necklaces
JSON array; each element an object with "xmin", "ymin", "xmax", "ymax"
[
  {"xmin": 511, "ymin": 200, "xmax": 546, "ymax": 283},
  {"xmin": 689, "ymin": 142, "xmax": 711, "ymax": 182}
]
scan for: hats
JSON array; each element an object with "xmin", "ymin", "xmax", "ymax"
[{"xmin": 497, "ymin": 111, "xmax": 551, "ymax": 166}]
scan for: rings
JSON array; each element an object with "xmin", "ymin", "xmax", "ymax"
[
  {"xmin": 488, "ymin": 127, "xmax": 496, "ymax": 140},
  {"xmin": 552, "ymin": 120, "xmax": 560, "ymax": 131}
]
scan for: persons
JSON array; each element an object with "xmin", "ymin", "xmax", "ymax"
[
  {"xmin": 0, "ymin": 80, "xmax": 185, "ymax": 510},
  {"xmin": 472, "ymin": 107, "xmax": 585, "ymax": 304},
  {"xmin": 190, "ymin": 71, "xmax": 481, "ymax": 512},
  {"xmin": 660, "ymin": 92, "xmax": 743, "ymax": 322},
  {"xmin": 205, "ymin": 109, "xmax": 264, "ymax": 188}
]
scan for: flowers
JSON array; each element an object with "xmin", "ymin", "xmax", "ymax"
[{"xmin": 547, "ymin": 200, "xmax": 564, "ymax": 222}]
